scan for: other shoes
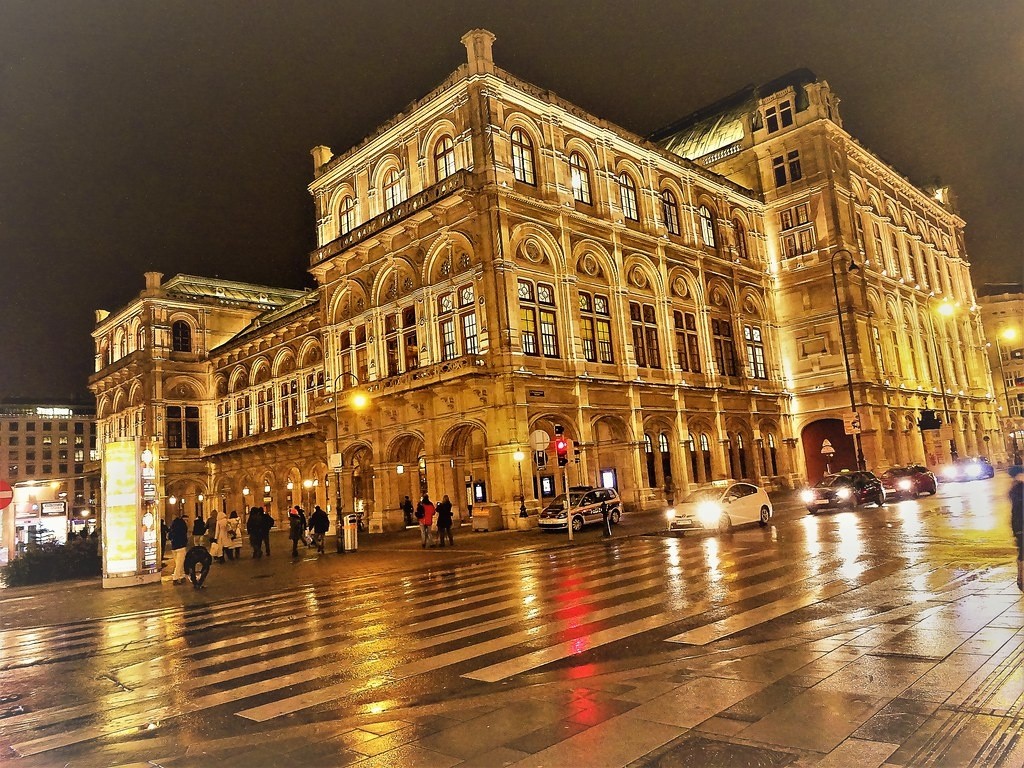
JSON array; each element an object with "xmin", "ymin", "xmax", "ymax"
[
  {"xmin": 318, "ymin": 545, "xmax": 325, "ymax": 555},
  {"xmin": 422, "ymin": 544, "xmax": 426, "ymax": 548},
  {"xmin": 198, "ymin": 585, "xmax": 205, "ymax": 589},
  {"xmin": 439, "ymin": 542, "xmax": 445, "ymax": 548},
  {"xmin": 430, "ymin": 545, "xmax": 437, "ymax": 547},
  {"xmin": 180, "ymin": 577, "xmax": 186, "ymax": 583},
  {"xmin": 173, "ymin": 579, "xmax": 181, "ymax": 586},
  {"xmin": 194, "ymin": 584, "xmax": 199, "ymax": 588}
]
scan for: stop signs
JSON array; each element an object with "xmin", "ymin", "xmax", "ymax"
[{"xmin": 0, "ymin": 480, "xmax": 13, "ymax": 510}]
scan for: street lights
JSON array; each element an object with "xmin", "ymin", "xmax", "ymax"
[
  {"xmin": 995, "ymin": 326, "xmax": 1022, "ymax": 465},
  {"xmin": 513, "ymin": 447, "xmax": 533, "ymax": 532},
  {"xmin": 830, "ymin": 249, "xmax": 867, "ymax": 472},
  {"xmin": 335, "ymin": 373, "xmax": 365, "ymax": 555},
  {"xmin": 927, "ymin": 293, "xmax": 959, "ymax": 462}
]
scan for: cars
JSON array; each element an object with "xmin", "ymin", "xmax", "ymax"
[
  {"xmin": 940, "ymin": 456, "xmax": 994, "ymax": 483},
  {"xmin": 806, "ymin": 469, "xmax": 886, "ymax": 514},
  {"xmin": 881, "ymin": 463, "xmax": 938, "ymax": 500},
  {"xmin": 667, "ymin": 482, "xmax": 774, "ymax": 538},
  {"xmin": 538, "ymin": 486, "xmax": 622, "ymax": 531}
]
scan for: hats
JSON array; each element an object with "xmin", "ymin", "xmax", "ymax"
[
  {"xmin": 315, "ymin": 506, "xmax": 320, "ymax": 509},
  {"xmin": 291, "ymin": 509, "xmax": 298, "ymax": 514}
]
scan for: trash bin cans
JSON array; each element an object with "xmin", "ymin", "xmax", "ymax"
[
  {"xmin": 344, "ymin": 514, "xmax": 358, "ymax": 550},
  {"xmin": 472, "ymin": 503, "xmax": 503, "ymax": 533},
  {"xmin": 770, "ymin": 475, "xmax": 790, "ymax": 491}
]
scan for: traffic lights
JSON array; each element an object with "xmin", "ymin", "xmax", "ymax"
[{"xmin": 556, "ymin": 439, "xmax": 569, "ymax": 469}]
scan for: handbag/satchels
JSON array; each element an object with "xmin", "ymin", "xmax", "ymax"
[
  {"xmin": 415, "ymin": 502, "xmax": 425, "ymax": 518},
  {"xmin": 306, "ymin": 534, "xmax": 314, "ymax": 545},
  {"xmin": 228, "ymin": 530, "xmax": 237, "ymax": 540},
  {"xmin": 209, "ymin": 541, "xmax": 222, "ymax": 557}
]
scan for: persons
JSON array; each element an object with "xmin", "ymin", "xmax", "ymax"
[
  {"xmin": 159, "ymin": 508, "xmax": 275, "ymax": 564},
  {"xmin": 183, "ymin": 546, "xmax": 212, "ymax": 589},
  {"xmin": 435, "ymin": 495, "xmax": 454, "ymax": 547},
  {"xmin": 168, "ymin": 517, "xmax": 189, "ymax": 586},
  {"xmin": 1008, "ymin": 465, "xmax": 1024, "ymax": 591},
  {"xmin": 415, "ymin": 494, "xmax": 436, "ymax": 547},
  {"xmin": 289, "ymin": 505, "xmax": 307, "ymax": 557},
  {"xmin": 308, "ymin": 506, "xmax": 330, "ymax": 553},
  {"xmin": 403, "ymin": 496, "xmax": 412, "ymax": 524}
]
